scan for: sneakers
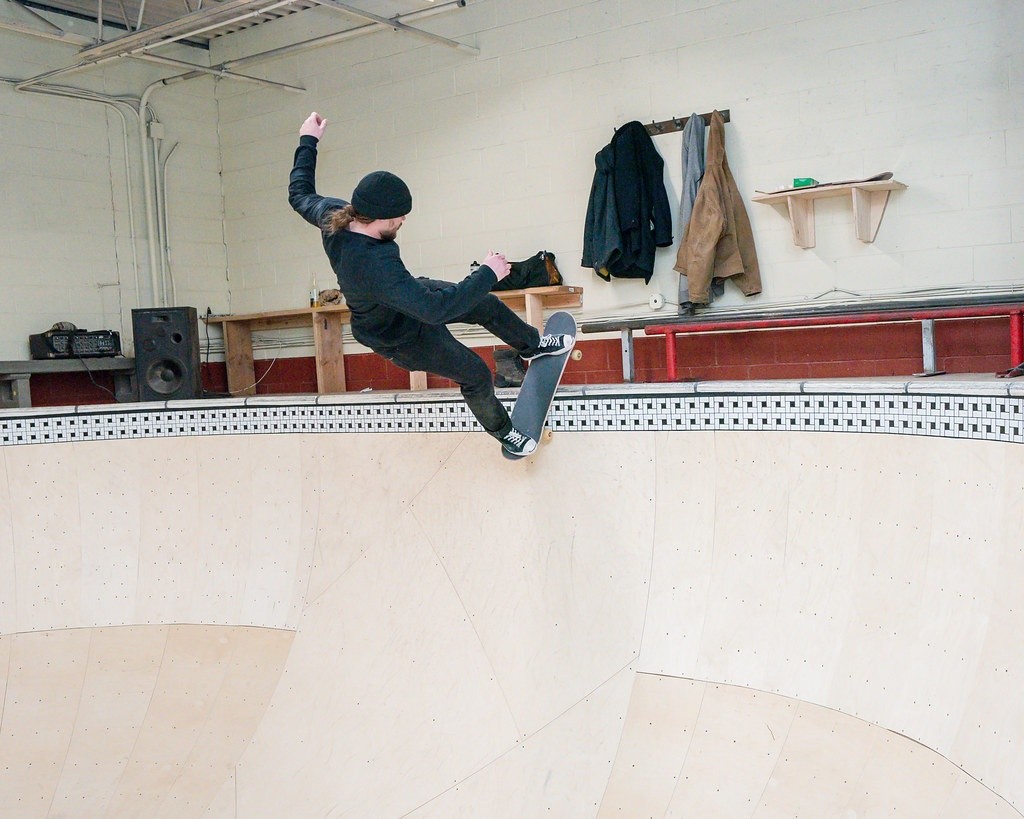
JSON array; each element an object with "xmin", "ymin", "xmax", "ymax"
[
  {"xmin": 520, "ymin": 333, "xmax": 575, "ymax": 361},
  {"xmin": 496, "ymin": 427, "xmax": 537, "ymax": 457}
]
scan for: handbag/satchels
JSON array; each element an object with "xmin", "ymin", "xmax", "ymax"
[{"xmin": 491, "ymin": 251, "xmax": 564, "ymax": 291}]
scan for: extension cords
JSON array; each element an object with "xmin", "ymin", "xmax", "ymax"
[{"xmin": 200, "ymin": 313, "xmax": 233, "ymax": 320}]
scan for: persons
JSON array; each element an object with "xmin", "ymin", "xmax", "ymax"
[{"xmin": 288, "ymin": 111, "xmax": 576, "ymax": 456}]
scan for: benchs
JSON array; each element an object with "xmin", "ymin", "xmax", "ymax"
[
  {"xmin": 582, "ymin": 293, "xmax": 1024, "ymax": 376},
  {"xmin": 201, "ymin": 285, "xmax": 584, "ymax": 396},
  {"xmin": 0, "ymin": 357, "xmax": 135, "ymax": 408}
]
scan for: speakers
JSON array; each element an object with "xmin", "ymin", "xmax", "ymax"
[{"xmin": 130, "ymin": 307, "xmax": 201, "ymax": 401}]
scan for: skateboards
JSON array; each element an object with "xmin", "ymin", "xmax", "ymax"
[{"xmin": 499, "ymin": 308, "xmax": 582, "ymax": 463}]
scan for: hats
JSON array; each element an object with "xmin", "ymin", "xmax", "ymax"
[{"xmin": 353, "ymin": 171, "xmax": 412, "ymax": 219}]
